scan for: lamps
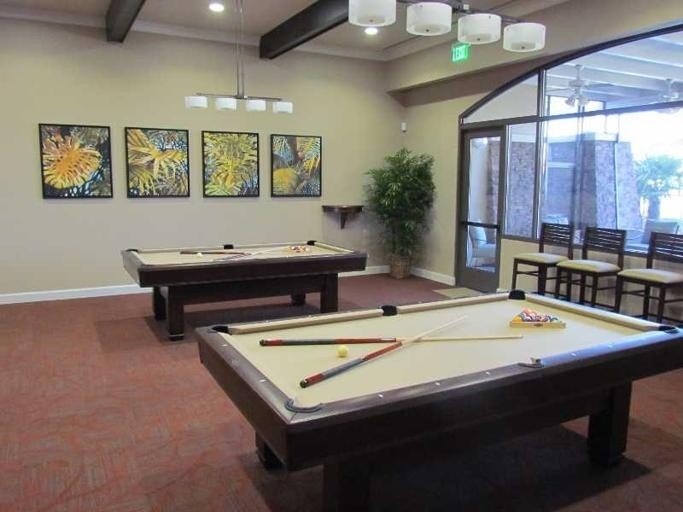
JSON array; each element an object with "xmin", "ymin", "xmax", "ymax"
[
  {"xmin": 185, "ymin": 0, "xmax": 294, "ymax": 115},
  {"xmin": 348, "ymin": 0, "xmax": 546, "ymax": 53},
  {"xmin": 565, "ymin": 92, "xmax": 590, "ymax": 106}
]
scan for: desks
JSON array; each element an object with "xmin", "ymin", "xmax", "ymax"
[
  {"xmin": 121, "ymin": 239, "xmax": 367, "ymax": 341},
  {"xmin": 195, "ymin": 290, "xmax": 683, "ymax": 512}
]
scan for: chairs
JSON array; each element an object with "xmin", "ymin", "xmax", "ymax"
[
  {"xmin": 613, "ymin": 231, "xmax": 683, "ymax": 323},
  {"xmin": 627, "ymin": 219, "xmax": 679, "ymax": 250},
  {"xmin": 511, "ymin": 221, "xmax": 574, "ymax": 302},
  {"xmin": 469, "ymin": 220, "xmax": 496, "ymax": 267},
  {"xmin": 555, "ymin": 226, "xmax": 626, "ymax": 312}
]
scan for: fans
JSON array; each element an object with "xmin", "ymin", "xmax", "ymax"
[{"xmin": 547, "ymin": 65, "xmax": 614, "ymax": 108}]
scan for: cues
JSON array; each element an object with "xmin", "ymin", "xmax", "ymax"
[
  {"xmin": 259, "ymin": 334, "xmax": 523, "ymax": 346},
  {"xmin": 300, "ymin": 316, "xmax": 466, "ymax": 388},
  {"xmin": 179, "ymin": 246, "xmax": 283, "ymax": 262}
]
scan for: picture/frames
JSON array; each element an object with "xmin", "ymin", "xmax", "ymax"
[
  {"xmin": 202, "ymin": 130, "xmax": 260, "ymax": 198},
  {"xmin": 270, "ymin": 133, "xmax": 322, "ymax": 198},
  {"xmin": 39, "ymin": 123, "xmax": 113, "ymax": 200},
  {"xmin": 125, "ymin": 127, "xmax": 191, "ymax": 198}
]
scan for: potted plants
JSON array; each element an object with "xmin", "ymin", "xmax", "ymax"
[{"xmin": 358, "ymin": 145, "xmax": 438, "ymax": 280}]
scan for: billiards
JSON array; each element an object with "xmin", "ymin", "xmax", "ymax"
[
  {"xmin": 285, "ymin": 244, "xmax": 310, "ymax": 253},
  {"xmin": 511, "ymin": 307, "xmax": 560, "ymax": 323},
  {"xmin": 336, "ymin": 344, "xmax": 349, "ymax": 356},
  {"xmin": 196, "ymin": 251, "xmax": 202, "ymax": 258}
]
